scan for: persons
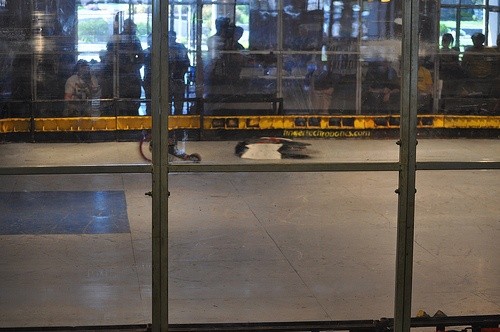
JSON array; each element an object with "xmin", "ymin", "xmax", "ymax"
[
  {"xmin": 0, "ymin": 18, "xmax": 500, "ymax": 143},
  {"xmin": 61, "ymin": 60, "xmax": 100, "ymax": 140}
]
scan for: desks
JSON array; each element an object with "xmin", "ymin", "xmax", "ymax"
[{"xmin": 240, "ymin": 69, "xmax": 306, "ymax": 114}]
